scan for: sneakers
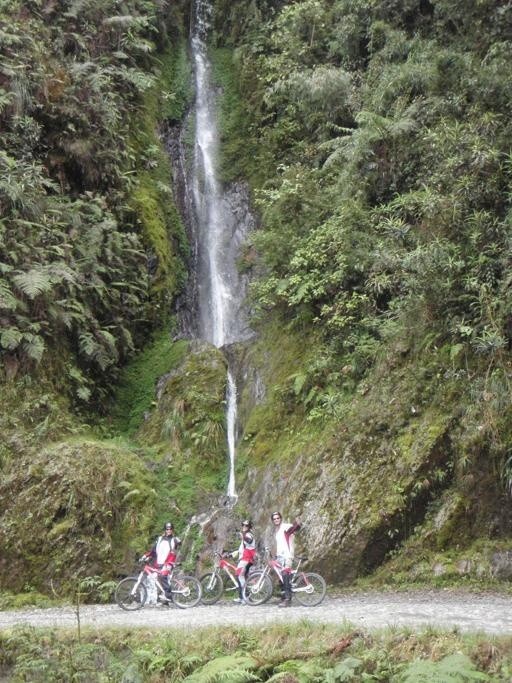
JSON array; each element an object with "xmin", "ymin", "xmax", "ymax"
[
  {"xmin": 156, "ymin": 595, "xmax": 172, "ymax": 607},
  {"xmin": 233, "ymin": 590, "xmax": 254, "ymax": 604},
  {"xmin": 278, "ymin": 599, "xmax": 292, "ymax": 607}
]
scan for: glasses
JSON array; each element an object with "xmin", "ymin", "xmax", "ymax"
[
  {"xmin": 167, "ymin": 528, "xmax": 172, "ymax": 530},
  {"xmin": 242, "ymin": 524, "xmax": 249, "ymax": 528}
]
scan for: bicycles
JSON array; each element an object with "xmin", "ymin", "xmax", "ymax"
[
  {"xmin": 199, "ymin": 552, "xmax": 273, "ymax": 605},
  {"xmin": 242, "ymin": 548, "xmax": 327, "ymax": 606},
  {"xmin": 114, "ymin": 555, "xmax": 204, "ymax": 611}
]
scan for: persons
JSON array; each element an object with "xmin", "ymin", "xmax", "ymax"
[
  {"xmin": 140, "ymin": 521, "xmax": 182, "ymax": 607},
  {"xmin": 223, "ymin": 520, "xmax": 256, "ymax": 605},
  {"xmin": 270, "ymin": 512, "xmax": 302, "ymax": 607}
]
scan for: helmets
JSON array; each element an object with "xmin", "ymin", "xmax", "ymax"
[
  {"xmin": 271, "ymin": 511, "xmax": 282, "ymax": 520},
  {"xmin": 241, "ymin": 520, "xmax": 253, "ymax": 529},
  {"xmin": 164, "ymin": 521, "xmax": 174, "ymax": 529}
]
